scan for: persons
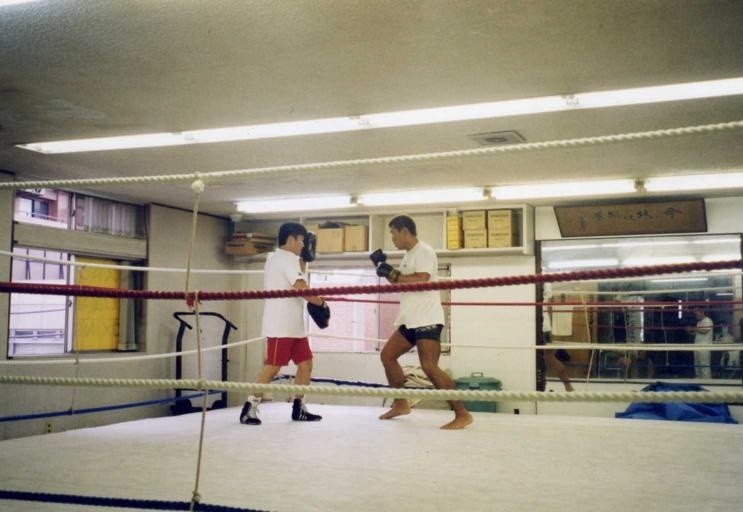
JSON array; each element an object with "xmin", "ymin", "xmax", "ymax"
[
  {"xmin": 685, "ymin": 306, "xmax": 713, "ymax": 378},
  {"xmin": 541, "ymin": 270, "xmax": 576, "ymax": 391},
  {"xmin": 369, "ymin": 215, "xmax": 473, "ymax": 431},
  {"xmin": 238, "ymin": 222, "xmax": 330, "ymax": 425}
]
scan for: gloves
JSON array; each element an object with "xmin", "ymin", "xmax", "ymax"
[
  {"xmin": 369, "ymin": 249, "xmax": 385, "ymax": 266},
  {"xmin": 376, "ymin": 263, "xmax": 400, "ymax": 281}
]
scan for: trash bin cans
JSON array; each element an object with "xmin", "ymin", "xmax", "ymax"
[{"xmin": 453, "ymin": 372, "xmax": 503, "ymax": 413}]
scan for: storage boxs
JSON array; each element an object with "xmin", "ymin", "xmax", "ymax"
[
  {"xmin": 304, "ymin": 223, "xmax": 322, "ymax": 252},
  {"xmin": 485, "ymin": 210, "xmax": 519, "ymax": 230},
  {"xmin": 460, "ymin": 210, "xmax": 487, "ymax": 230},
  {"xmin": 463, "ymin": 230, "xmax": 487, "ymax": 248},
  {"xmin": 316, "ymin": 227, "xmax": 344, "ymax": 253},
  {"xmin": 343, "ymin": 225, "xmax": 368, "ymax": 250},
  {"xmin": 487, "ymin": 230, "xmax": 519, "ymax": 247}
]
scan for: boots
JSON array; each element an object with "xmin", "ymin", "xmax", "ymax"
[
  {"xmin": 291, "ymin": 399, "xmax": 321, "ymax": 420},
  {"xmin": 239, "ymin": 395, "xmax": 261, "ymax": 425}
]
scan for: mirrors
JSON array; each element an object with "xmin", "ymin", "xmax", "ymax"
[{"xmin": 532, "ymin": 233, "xmax": 741, "ymax": 391}]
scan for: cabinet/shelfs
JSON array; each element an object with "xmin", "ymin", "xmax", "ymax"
[{"xmin": 226, "ymin": 202, "xmax": 536, "ymax": 256}]
